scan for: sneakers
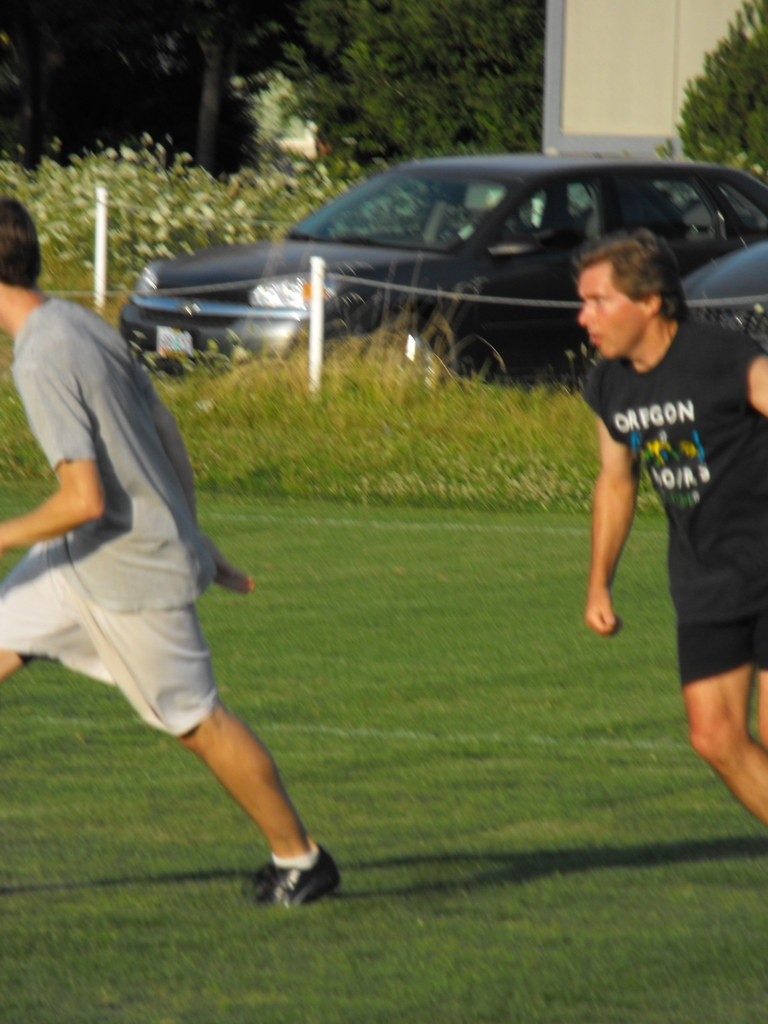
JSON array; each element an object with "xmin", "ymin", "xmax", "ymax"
[{"xmin": 243, "ymin": 834, "xmax": 340, "ymax": 908}]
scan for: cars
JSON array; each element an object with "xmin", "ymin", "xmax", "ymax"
[
  {"xmin": 677, "ymin": 230, "xmax": 768, "ymax": 356},
  {"xmin": 114, "ymin": 153, "xmax": 767, "ymax": 389}
]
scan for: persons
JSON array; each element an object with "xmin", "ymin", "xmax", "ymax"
[
  {"xmin": 577, "ymin": 229, "xmax": 768, "ymax": 823},
  {"xmin": 1, "ymin": 190, "xmax": 342, "ymax": 906}
]
